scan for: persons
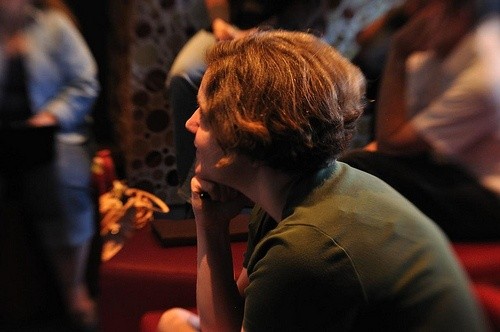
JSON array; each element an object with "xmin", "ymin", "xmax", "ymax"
[
  {"xmin": 0, "ymin": 0, "xmax": 101, "ymax": 332},
  {"xmin": 158, "ymin": 31, "xmax": 488, "ymax": 332},
  {"xmin": 164, "ymin": 0, "xmax": 500, "ymax": 245}
]
